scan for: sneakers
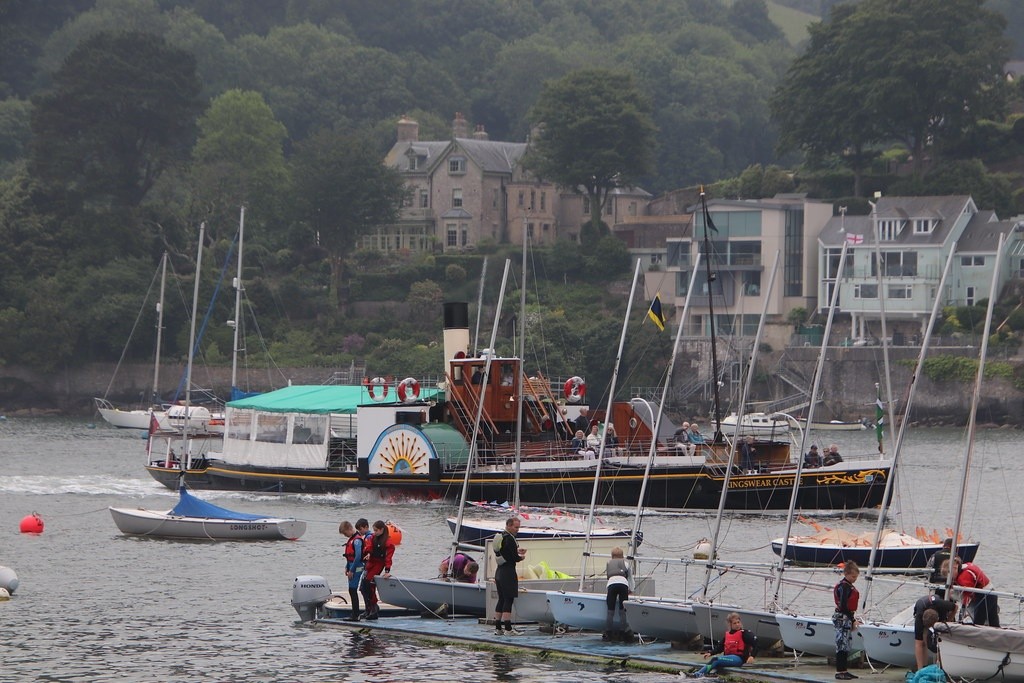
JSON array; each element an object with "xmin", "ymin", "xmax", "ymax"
[
  {"xmin": 503, "ymin": 629, "xmax": 524, "ymax": 636},
  {"xmin": 495, "ymin": 629, "xmax": 504, "ymax": 635}
]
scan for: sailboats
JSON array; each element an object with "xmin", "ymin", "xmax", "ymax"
[
  {"xmin": 374, "ymin": 231, "xmax": 1023, "ymax": 683},
  {"xmin": 94, "ymin": 206, "xmax": 277, "ymax": 435},
  {"xmin": 107, "ymin": 222, "xmax": 308, "ymax": 540}
]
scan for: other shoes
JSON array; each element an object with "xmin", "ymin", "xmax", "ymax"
[
  {"xmin": 683, "ymin": 672, "xmax": 696, "ymax": 678},
  {"xmin": 619, "ymin": 630, "xmax": 625, "ymax": 641},
  {"xmin": 359, "ymin": 610, "xmax": 369, "ymax": 618},
  {"xmin": 835, "ymin": 672, "xmax": 858, "ymax": 680},
  {"xmin": 603, "ymin": 631, "xmax": 612, "ymax": 641},
  {"xmin": 343, "ymin": 614, "xmax": 360, "ymax": 622},
  {"xmin": 366, "ymin": 611, "xmax": 378, "ymax": 620}
]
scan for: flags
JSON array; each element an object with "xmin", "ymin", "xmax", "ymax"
[
  {"xmin": 647, "ymin": 290, "xmax": 665, "ymax": 333},
  {"xmin": 846, "ymin": 232, "xmax": 864, "ymax": 245},
  {"xmin": 875, "ymin": 390, "xmax": 883, "ymax": 454}
]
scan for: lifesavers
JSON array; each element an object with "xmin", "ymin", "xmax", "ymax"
[
  {"xmin": 368, "ymin": 377, "xmax": 388, "ymax": 402},
  {"xmin": 397, "ymin": 378, "xmax": 420, "ymax": 404},
  {"xmin": 563, "ymin": 376, "xmax": 586, "ymax": 403}
]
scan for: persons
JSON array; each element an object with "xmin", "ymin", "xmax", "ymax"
[
  {"xmin": 670, "ymin": 422, "xmax": 692, "ymax": 456},
  {"xmin": 688, "ymin": 423, "xmax": 706, "ymax": 444},
  {"xmin": 913, "ymin": 594, "xmax": 958, "ymax": 672},
  {"xmin": 831, "ymin": 558, "xmax": 860, "ymax": 681},
  {"xmin": 605, "ymin": 427, "xmax": 623, "ymax": 457},
  {"xmin": 439, "ymin": 552, "xmax": 479, "ymax": 584},
  {"xmin": 586, "ymin": 425, "xmax": 611, "ymax": 458},
  {"xmin": 501, "ymin": 367, "xmax": 514, "ymax": 386},
  {"xmin": 471, "ymin": 366, "xmax": 491, "ymax": 384},
  {"xmin": 356, "ymin": 518, "xmax": 395, "ymax": 620},
  {"xmin": 741, "ymin": 435, "xmax": 756, "ymax": 473},
  {"xmin": 602, "ymin": 547, "xmax": 635, "ymax": 643},
  {"xmin": 924, "ymin": 537, "xmax": 962, "ymax": 622},
  {"xmin": 493, "ymin": 517, "xmax": 527, "ymax": 636},
  {"xmin": 822, "ymin": 448, "xmax": 836, "ymax": 466},
  {"xmin": 571, "ymin": 430, "xmax": 595, "ymax": 460},
  {"xmin": 804, "ymin": 444, "xmax": 824, "ymax": 469},
  {"xmin": 339, "ymin": 520, "xmax": 368, "ymax": 621},
  {"xmin": 941, "ymin": 555, "xmax": 1001, "ymax": 628},
  {"xmin": 574, "ymin": 407, "xmax": 592, "ymax": 437},
  {"xmin": 683, "ymin": 612, "xmax": 757, "ymax": 679},
  {"xmin": 830, "ymin": 444, "xmax": 843, "ymax": 463}
]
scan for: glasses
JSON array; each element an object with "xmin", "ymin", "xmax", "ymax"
[{"xmin": 823, "ymin": 451, "xmax": 829, "ymax": 453}]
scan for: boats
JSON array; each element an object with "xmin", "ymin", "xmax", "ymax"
[
  {"xmin": 787, "ymin": 416, "xmax": 861, "ymax": 430},
  {"xmin": 146, "ymin": 184, "xmax": 900, "ymax": 510},
  {"xmin": 711, "ymin": 412, "xmax": 789, "ymax": 436}
]
